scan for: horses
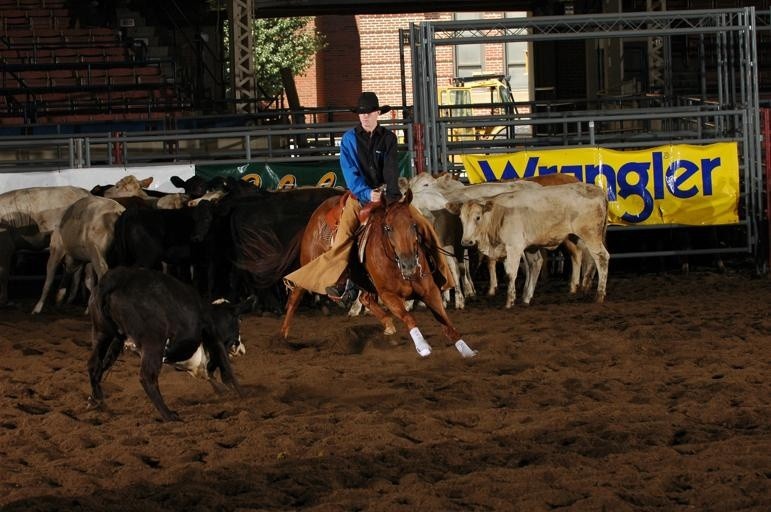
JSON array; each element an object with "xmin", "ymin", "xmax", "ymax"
[{"xmin": 230, "ymin": 186, "xmax": 479, "ymax": 360}]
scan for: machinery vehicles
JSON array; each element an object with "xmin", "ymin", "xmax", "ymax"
[{"xmin": 396, "ymin": 74, "xmax": 534, "ymax": 163}]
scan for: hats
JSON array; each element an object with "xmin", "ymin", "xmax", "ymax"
[{"xmin": 348, "ymin": 92, "xmax": 392, "ymax": 115}]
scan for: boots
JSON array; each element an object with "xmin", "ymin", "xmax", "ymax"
[{"xmin": 325, "ymin": 287, "xmax": 352, "ymax": 312}]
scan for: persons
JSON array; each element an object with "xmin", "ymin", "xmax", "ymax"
[{"xmin": 325, "ymin": 91, "xmax": 412, "ymax": 310}]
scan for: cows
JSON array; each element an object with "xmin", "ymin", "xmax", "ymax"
[
  {"xmin": 0, "ymin": 172, "xmax": 611, "ymax": 316},
  {"xmin": 85, "ymin": 265, "xmax": 259, "ymax": 422}
]
